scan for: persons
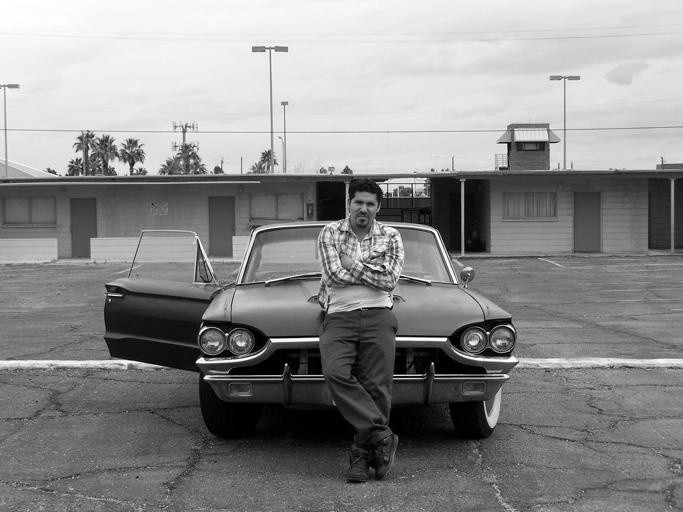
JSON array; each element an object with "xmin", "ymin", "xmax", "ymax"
[{"xmin": 316, "ymin": 178, "xmax": 404, "ymax": 484}]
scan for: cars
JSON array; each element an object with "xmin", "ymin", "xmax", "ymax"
[{"xmin": 102, "ymin": 218, "xmax": 519, "ymax": 442}]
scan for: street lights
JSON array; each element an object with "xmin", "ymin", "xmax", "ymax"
[
  {"xmin": 172, "ymin": 119, "xmax": 199, "ymax": 176},
  {"xmin": 548, "ymin": 72, "xmax": 579, "ymax": 171},
  {"xmin": 251, "ymin": 44, "xmax": 289, "ymax": 176},
  {"xmin": 276, "ymin": 99, "xmax": 288, "ymax": 176},
  {"xmin": 0, "ymin": 81, "xmax": 21, "ymax": 179}
]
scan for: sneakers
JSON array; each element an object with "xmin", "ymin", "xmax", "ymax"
[
  {"xmin": 370, "ymin": 430, "xmax": 400, "ymax": 480},
  {"xmin": 347, "ymin": 442, "xmax": 369, "ymax": 483}
]
